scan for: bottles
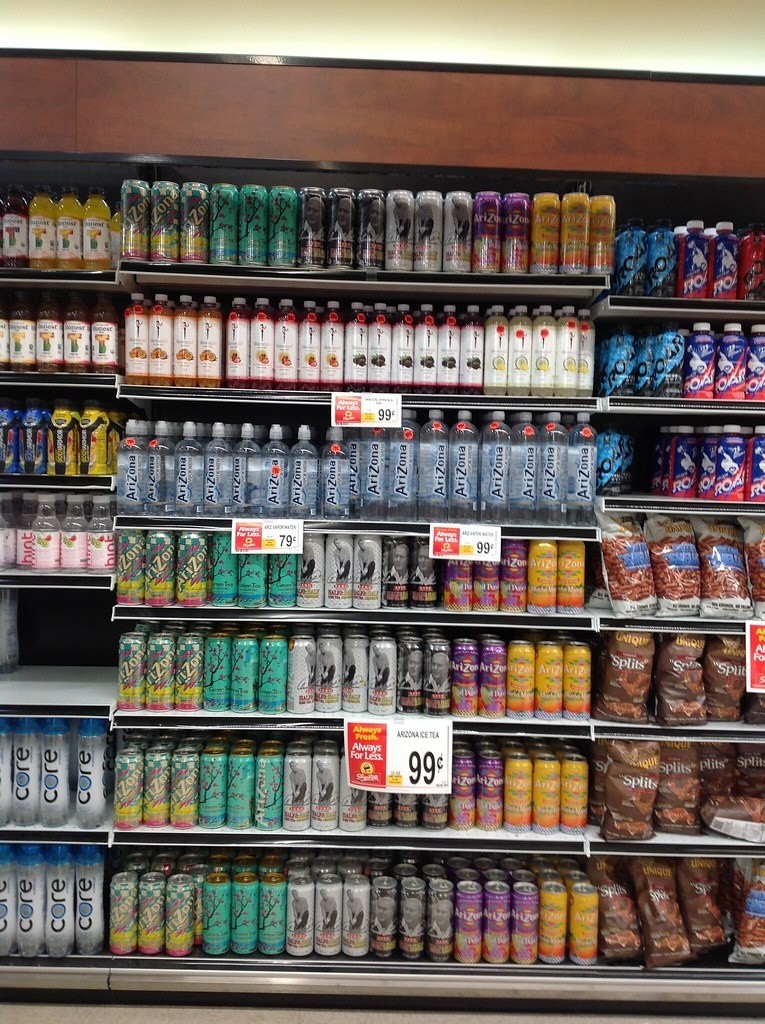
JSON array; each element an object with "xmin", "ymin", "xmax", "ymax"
[
  {"xmin": 0, "ymin": 396, "xmax": 117, "ymax": 474},
  {"xmin": 595, "ymin": 322, "xmax": 765, "ymax": 400},
  {"xmin": 0, "ymin": 291, "xmax": 125, "ymax": 376},
  {"xmin": 363, "ymin": 410, "xmax": 596, "ymax": 527},
  {"xmin": 0, "ymin": 491, "xmax": 117, "ymax": 574},
  {"xmin": 616, "ymin": 218, "xmax": 765, "ymax": 301},
  {"xmin": 76, "ymin": 719, "xmax": 106, "ymax": 828},
  {"xmin": 76, "ymin": 843, "xmax": 103, "ymax": 954},
  {"xmin": 596, "ymin": 425, "xmax": 765, "ymax": 503},
  {"xmin": 0, "ymin": 844, "xmax": 16, "ymax": 956},
  {"xmin": 0, "ymin": 576, "xmax": 19, "ymax": 673},
  {"xmin": 46, "ymin": 844, "xmax": 75, "ymax": 958},
  {"xmin": 117, "ymin": 419, "xmax": 362, "ymax": 520},
  {"xmin": 41, "ymin": 718, "xmax": 70, "ymax": 827},
  {"xmin": 13, "ymin": 717, "xmax": 41, "ymax": 827},
  {"xmin": 126, "ymin": 293, "xmax": 343, "ymax": 392},
  {"xmin": 0, "ymin": 717, "xmax": 12, "ymax": 829},
  {"xmin": 16, "ymin": 845, "xmax": 45, "ymax": 957},
  {"xmin": 0, "ymin": 184, "xmax": 122, "ymax": 269},
  {"xmin": 343, "ymin": 303, "xmax": 594, "ymax": 397}
]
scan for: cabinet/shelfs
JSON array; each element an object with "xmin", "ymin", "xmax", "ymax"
[
  {"xmin": 602, "ymin": 169, "xmax": 762, "ymax": 1014},
  {"xmin": 0, "ymin": 153, "xmax": 111, "ymax": 1005},
  {"xmin": 111, "ymin": 157, "xmax": 600, "ymax": 1005}
]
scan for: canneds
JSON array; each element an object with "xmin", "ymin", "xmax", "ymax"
[
  {"xmin": 115, "ymin": 530, "xmax": 585, "ymax": 614},
  {"xmin": 117, "ymin": 620, "xmax": 591, "ymax": 722},
  {"xmin": 119, "ymin": 178, "xmax": 615, "ymax": 275},
  {"xmin": 109, "ymin": 847, "xmax": 600, "ymax": 967},
  {"xmin": 113, "ymin": 731, "xmax": 588, "ymax": 836}
]
